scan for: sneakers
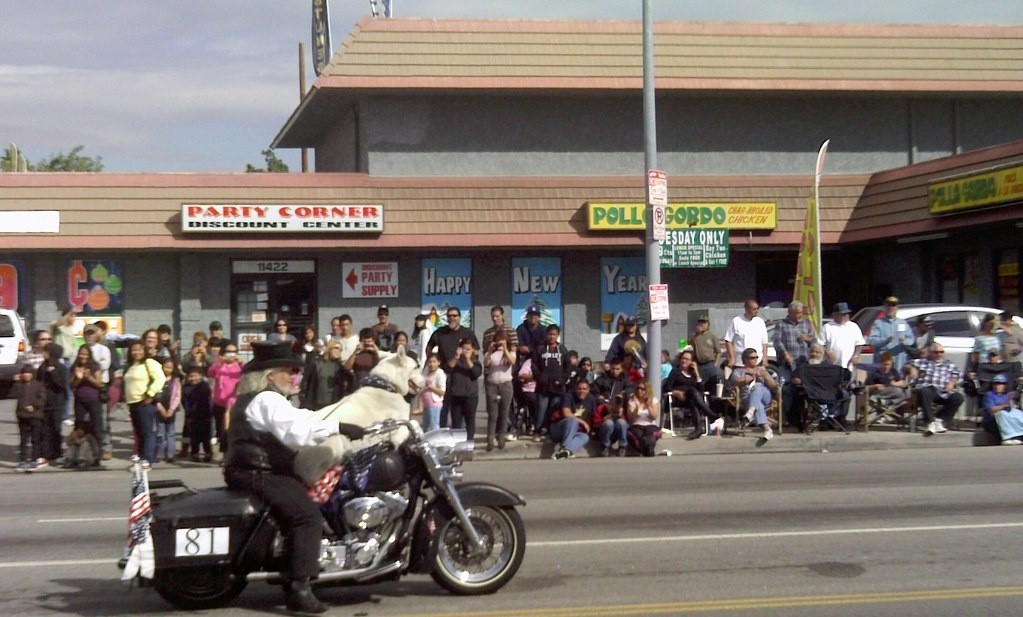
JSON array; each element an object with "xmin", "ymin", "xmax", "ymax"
[
  {"xmin": 285, "ymin": 578, "xmax": 328, "ymax": 613},
  {"xmin": 924, "ymin": 417, "xmax": 947, "ymax": 435}
]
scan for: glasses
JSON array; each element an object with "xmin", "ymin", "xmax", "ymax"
[
  {"xmin": 446, "ymin": 314, "xmax": 459, "ymax": 318},
  {"xmin": 932, "ymin": 350, "xmax": 944, "ymax": 354},
  {"xmin": 277, "ymin": 324, "xmax": 287, "ymax": 326}
]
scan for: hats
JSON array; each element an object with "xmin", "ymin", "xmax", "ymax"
[
  {"xmin": 624, "ymin": 315, "xmax": 637, "ymax": 325},
  {"xmin": 83, "ymin": 324, "xmax": 97, "ymax": 333},
  {"xmin": 831, "ymin": 303, "xmax": 851, "ymax": 314},
  {"xmin": 697, "ymin": 315, "xmax": 709, "ymax": 322},
  {"xmin": 527, "ymin": 305, "xmax": 541, "ymax": 315},
  {"xmin": 241, "ymin": 339, "xmax": 305, "ymax": 373},
  {"xmin": 883, "ymin": 296, "xmax": 900, "ymax": 306},
  {"xmin": 378, "ymin": 304, "xmax": 389, "ymax": 312},
  {"xmin": 987, "ymin": 311, "xmax": 1012, "ymax": 383},
  {"xmin": 918, "ymin": 314, "xmax": 935, "ymax": 325}
]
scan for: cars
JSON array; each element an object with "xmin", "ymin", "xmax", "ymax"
[{"xmin": 718, "ymin": 317, "xmax": 834, "ymax": 386}]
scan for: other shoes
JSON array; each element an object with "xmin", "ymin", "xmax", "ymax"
[
  {"xmin": 14, "ymin": 446, "xmax": 213, "ymax": 472},
  {"xmin": 487, "ymin": 425, "xmax": 547, "ymax": 452},
  {"xmin": 555, "ymin": 445, "xmax": 655, "ymax": 458},
  {"xmin": 744, "ymin": 409, "xmax": 774, "ymax": 441}
]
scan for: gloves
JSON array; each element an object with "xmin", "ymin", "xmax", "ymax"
[{"xmin": 339, "ymin": 422, "xmax": 366, "ymax": 441}]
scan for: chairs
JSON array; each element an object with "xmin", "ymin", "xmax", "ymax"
[{"xmin": 655, "ymin": 364, "xmax": 920, "ymax": 436}]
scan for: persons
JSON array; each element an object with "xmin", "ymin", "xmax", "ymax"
[
  {"xmin": 11, "ymin": 297, "xmax": 1023, "ymax": 482},
  {"xmin": 223, "ymin": 340, "xmax": 365, "ymax": 613}
]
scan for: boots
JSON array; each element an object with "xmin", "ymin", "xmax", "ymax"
[{"xmin": 687, "ymin": 393, "xmax": 720, "ymax": 441}]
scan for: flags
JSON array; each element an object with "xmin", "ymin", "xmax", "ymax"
[{"xmin": 116, "ymin": 472, "xmax": 157, "ymax": 576}]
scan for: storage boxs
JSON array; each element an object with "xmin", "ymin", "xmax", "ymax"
[{"xmin": 152, "ymin": 483, "xmax": 268, "ymax": 570}]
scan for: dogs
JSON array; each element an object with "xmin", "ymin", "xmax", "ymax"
[{"xmin": 294, "ymin": 345, "xmax": 426, "ymax": 485}]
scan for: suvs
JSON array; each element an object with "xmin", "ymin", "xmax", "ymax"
[
  {"xmin": 849, "ymin": 303, "xmax": 1023, "ymax": 384},
  {"xmin": 0, "ymin": 308, "xmax": 26, "ymax": 378}
]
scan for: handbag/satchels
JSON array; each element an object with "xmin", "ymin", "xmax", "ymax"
[{"xmin": 144, "ymin": 356, "xmax": 169, "ymax": 402}]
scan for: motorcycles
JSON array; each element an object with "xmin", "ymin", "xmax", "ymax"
[{"xmin": 116, "ymin": 417, "xmax": 526, "ymax": 611}]
